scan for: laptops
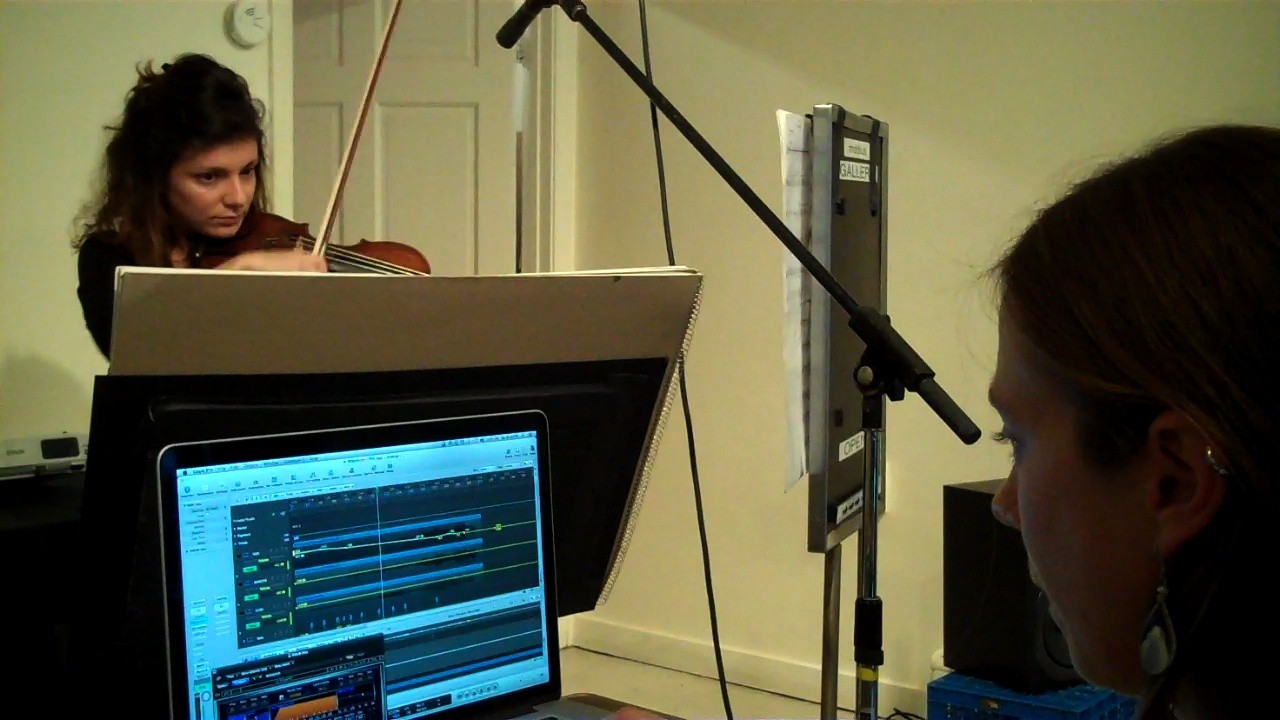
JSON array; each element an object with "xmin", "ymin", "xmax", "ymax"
[{"xmin": 158, "ymin": 409, "xmax": 689, "ymax": 720}]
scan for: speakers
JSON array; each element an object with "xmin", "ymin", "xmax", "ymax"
[{"xmin": 942, "ymin": 477, "xmax": 1088, "ymax": 695}]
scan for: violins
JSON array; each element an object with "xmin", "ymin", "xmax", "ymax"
[{"xmin": 187, "ymin": 211, "xmax": 431, "ymax": 276}]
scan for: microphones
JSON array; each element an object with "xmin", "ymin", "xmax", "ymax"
[{"xmin": 496, "ymin": 0, "xmax": 548, "ymax": 49}]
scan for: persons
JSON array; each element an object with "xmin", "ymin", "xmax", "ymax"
[
  {"xmin": 987, "ymin": 121, "xmax": 1280, "ymax": 720},
  {"xmin": 69, "ymin": 52, "xmax": 327, "ymax": 363}
]
